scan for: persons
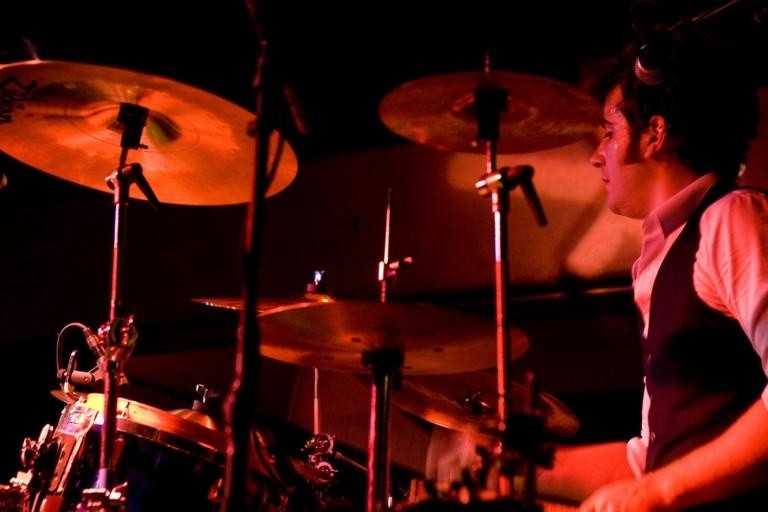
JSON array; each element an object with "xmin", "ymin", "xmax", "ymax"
[{"xmin": 422, "ymin": 25, "xmax": 768, "ymax": 512}]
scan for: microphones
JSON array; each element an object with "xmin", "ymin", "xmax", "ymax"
[
  {"xmin": 633, "ymin": 55, "xmax": 668, "ymax": 86},
  {"xmin": 82, "ymin": 326, "xmax": 129, "ymax": 386}
]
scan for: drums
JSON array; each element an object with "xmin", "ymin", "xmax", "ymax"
[{"xmin": 20, "ymin": 390, "xmax": 231, "ymax": 512}]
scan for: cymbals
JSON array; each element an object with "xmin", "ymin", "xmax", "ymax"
[
  {"xmin": 253, "ymin": 298, "xmax": 531, "ymax": 377},
  {"xmin": 366, "ymin": 370, "xmax": 579, "ymax": 438},
  {"xmin": 193, "ymin": 295, "xmax": 337, "ymax": 322},
  {"xmin": 379, "ymin": 69, "xmax": 608, "ymax": 155},
  {"xmin": 0, "ymin": 59, "xmax": 302, "ymax": 208}
]
